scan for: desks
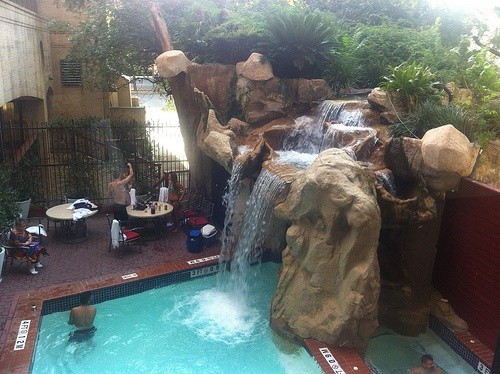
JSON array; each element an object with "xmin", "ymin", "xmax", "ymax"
[
  {"xmin": 45, "ymin": 202, "xmax": 99, "ymax": 246},
  {"xmin": 125, "ymin": 201, "xmax": 174, "ymax": 241}
]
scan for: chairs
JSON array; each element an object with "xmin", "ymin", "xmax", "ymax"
[
  {"xmin": 181, "ymin": 193, "xmax": 207, "ymax": 223},
  {"xmin": 104, "ymin": 213, "xmax": 143, "ymax": 260},
  {"xmin": 43, "ymin": 199, "xmax": 65, "ymax": 231},
  {"xmin": 188, "ymin": 201, "xmax": 215, "ymax": 229},
  {"xmin": 2, "ymin": 228, "xmax": 38, "ymax": 275}
]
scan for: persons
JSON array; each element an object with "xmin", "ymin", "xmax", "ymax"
[
  {"xmin": 163, "ymin": 172, "xmax": 186, "ymax": 233},
  {"xmin": 8, "ymin": 218, "xmax": 43, "ymax": 275},
  {"xmin": 108, "ymin": 163, "xmax": 134, "ymax": 228},
  {"xmin": 407, "ymin": 355, "xmax": 444, "ymax": 374},
  {"xmin": 68, "ymin": 292, "xmax": 98, "ymax": 341}
]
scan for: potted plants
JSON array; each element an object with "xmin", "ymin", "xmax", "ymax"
[{"xmin": 0, "ymin": 167, "xmax": 32, "ymax": 282}]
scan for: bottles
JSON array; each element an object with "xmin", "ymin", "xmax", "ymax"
[
  {"xmin": 165, "ymin": 205, "xmax": 167, "ymax": 210},
  {"xmin": 151, "ymin": 206, "xmax": 155, "ymax": 214}
]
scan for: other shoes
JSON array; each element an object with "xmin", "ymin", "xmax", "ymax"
[
  {"xmin": 29, "ymin": 268, "xmax": 39, "ymax": 274},
  {"xmin": 36, "ymin": 261, "xmax": 44, "ymax": 267}
]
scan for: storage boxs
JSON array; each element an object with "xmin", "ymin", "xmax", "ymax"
[{"xmin": 202, "ymin": 223, "xmax": 218, "ymax": 248}]
